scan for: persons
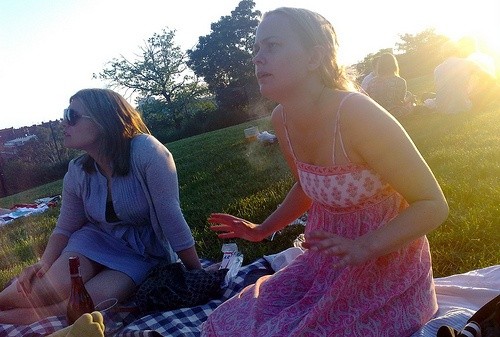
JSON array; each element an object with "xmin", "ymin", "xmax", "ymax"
[
  {"xmin": 422, "ymin": 32, "xmax": 480, "ymax": 110},
  {"xmin": 361, "ymin": 57, "xmax": 377, "ymax": 93},
  {"xmin": 366, "ymin": 54, "xmax": 414, "ymax": 118},
  {"xmin": 0, "ymin": 88, "xmax": 222, "ymax": 325},
  {"xmin": 202, "ymin": 6, "xmax": 449, "ymax": 337}
]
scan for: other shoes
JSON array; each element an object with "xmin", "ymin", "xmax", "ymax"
[{"xmin": 436, "ymin": 325, "xmax": 455, "ymax": 337}]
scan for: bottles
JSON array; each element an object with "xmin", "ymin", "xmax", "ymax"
[{"xmin": 66, "ymin": 256, "xmax": 95, "ymax": 326}]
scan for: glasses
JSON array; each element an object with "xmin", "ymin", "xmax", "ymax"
[{"xmin": 64, "ymin": 109, "xmax": 91, "ymax": 126}]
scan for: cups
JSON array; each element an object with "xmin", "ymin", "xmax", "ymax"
[{"xmin": 94, "ymin": 298, "xmax": 123, "ymax": 337}]
